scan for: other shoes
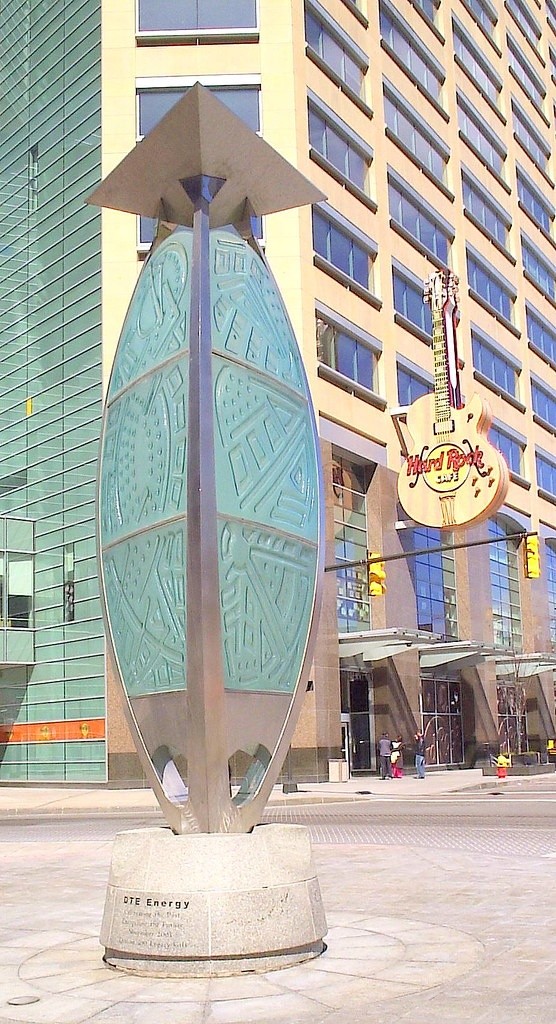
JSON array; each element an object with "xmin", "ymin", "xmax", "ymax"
[
  {"xmin": 379, "ymin": 778, "xmax": 385, "ymax": 780},
  {"xmin": 414, "ymin": 776, "xmax": 424, "ymax": 779}
]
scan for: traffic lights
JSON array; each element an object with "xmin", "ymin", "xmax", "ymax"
[{"xmin": 368, "ymin": 552, "xmax": 386, "ymax": 596}]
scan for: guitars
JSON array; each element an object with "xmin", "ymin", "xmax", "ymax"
[{"xmin": 397, "ymin": 268, "xmax": 509, "ymax": 532}]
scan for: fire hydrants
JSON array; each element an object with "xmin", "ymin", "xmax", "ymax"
[{"xmin": 497, "ymin": 754, "xmax": 510, "ymax": 779}]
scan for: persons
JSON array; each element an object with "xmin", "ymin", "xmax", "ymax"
[{"xmin": 377, "ymin": 729, "xmax": 425, "ymax": 780}]
